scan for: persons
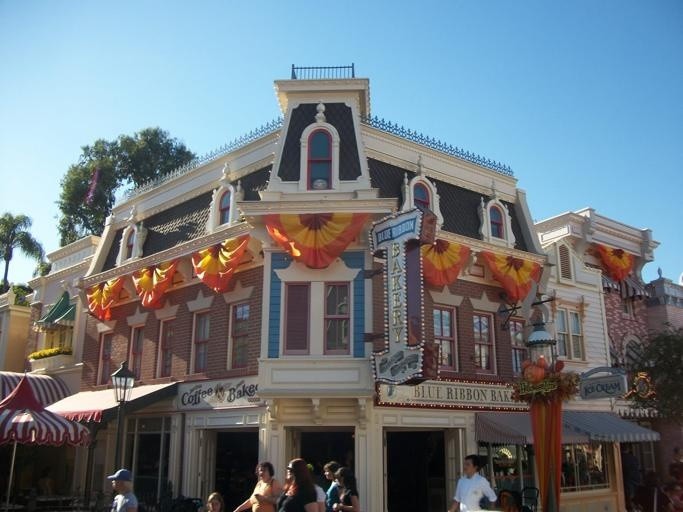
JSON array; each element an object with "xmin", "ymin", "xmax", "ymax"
[
  {"xmin": 321, "ymin": 461, "xmax": 342, "ymax": 512},
  {"xmin": 232, "ymin": 462, "xmax": 282, "ymax": 512},
  {"xmin": 37, "ymin": 466, "xmax": 56, "ymax": 496},
  {"xmin": 205, "ymin": 492, "xmax": 224, "ymax": 512},
  {"xmin": 104, "ymin": 467, "xmax": 140, "ymax": 512},
  {"xmin": 306, "ymin": 463, "xmax": 327, "ymax": 512},
  {"xmin": 274, "ymin": 458, "xmax": 319, "ymax": 511},
  {"xmin": 331, "ymin": 466, "xmax": 360, "ymax": 512},
  {"xmin": 663, "ymin": 482, "xmax": 682, "ymax": 511},
  {"xmin": 446, "ymin": 454, "xmax": 498, "ymax": 512},
  {"xmin": 619, "ymin": 442, "xmax": 644, "ymax": 512},
  {"xmin": 659, "ymin": 446, "xmax": 682, "ymax": 512}
]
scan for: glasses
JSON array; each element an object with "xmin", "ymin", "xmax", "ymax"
[{"xmin": 286, "ymin": 467, "xmax": 295, "ymax": 471}]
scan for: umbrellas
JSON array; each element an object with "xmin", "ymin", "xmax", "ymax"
[{"xmin": 0, "ymin": 368, "xmax": 93, "ymax": 512}]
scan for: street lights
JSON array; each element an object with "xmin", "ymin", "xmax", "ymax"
[{"xmin": 111, "ymin": 359, "xmax": 138, "ymax": 501}]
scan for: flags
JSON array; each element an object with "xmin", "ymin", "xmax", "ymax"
[{"xmin": 82, "ymin": 164, "xmax": 101, "ymax": 206}]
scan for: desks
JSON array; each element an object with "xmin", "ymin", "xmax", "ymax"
[{"xmin": 25, "ymin": 494, "xmax": 72, "ymax": 512}]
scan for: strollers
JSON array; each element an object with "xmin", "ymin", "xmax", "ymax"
[{"xmin": 498, "ymin": 485, "xmax": 540, "ymax": 512}]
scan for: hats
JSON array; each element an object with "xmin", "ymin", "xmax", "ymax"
[{"xmin": 107, "ymin": 469, "xmax": 134, "ymax": 480}]
